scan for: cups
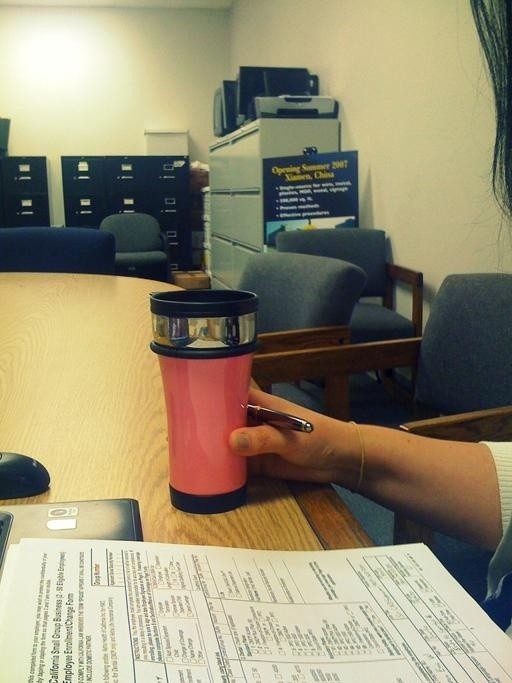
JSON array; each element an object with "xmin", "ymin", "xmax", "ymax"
[{"xmin": 150, "ymin": 289, "xmax": 259, "ymax": 514}]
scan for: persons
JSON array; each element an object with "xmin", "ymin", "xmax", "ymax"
[{"xmin": 228, "ymin": 0, "xmax": 511, "ymax": 645}]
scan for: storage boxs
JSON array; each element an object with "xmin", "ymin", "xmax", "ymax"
[{"xmin": 170, "ymin": 270, "xmax": 211, "ymax": 290}]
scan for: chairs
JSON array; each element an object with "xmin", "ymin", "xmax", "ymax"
[
  {"xmin": 275, "ymin": 228, "xmax": 424, "ymax": 406},
  {"xmin": 100, "ymin": 212, "xmax": 169, "ymax": 281},
  {"xmin": 252, "ymin": 271, "xmax": 512, "ymax": 544},
  {"xmin": 236, "ymin": 253, "xmax": 368, "ymax": 421},
  {"xmin": 0, "ymin": 226, "xmax": 116, "ymax": 274}
]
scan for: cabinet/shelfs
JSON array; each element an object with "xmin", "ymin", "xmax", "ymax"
[
  {"xmin": 207, "ymin": 118, "xmax": 340, "ymax": 291},
  {"xmin": 0, "ymin": 156, "xmax": 49, "ymax": 227},
  {"xmin": 61, "ymin": 155, "xmax": 190, "ymax": 271}
]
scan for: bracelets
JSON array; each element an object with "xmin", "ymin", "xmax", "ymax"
[{"xmin": 349, "ymin": 420, "xmax": 364, "ymax": 493}]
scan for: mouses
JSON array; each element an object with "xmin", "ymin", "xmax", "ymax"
[{"xmin": 1, "ymin": 453, "xmax": 51, "ymax": 500}]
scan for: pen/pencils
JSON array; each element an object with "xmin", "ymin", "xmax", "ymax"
[{"xmin": 247, "ymin": 405, "xmax": 313, "ymax": 436}]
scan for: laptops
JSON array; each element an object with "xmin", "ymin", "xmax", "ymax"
[{"xmin": 1, "ymin": 493, "xmax": 142, "ymax": 580}]
